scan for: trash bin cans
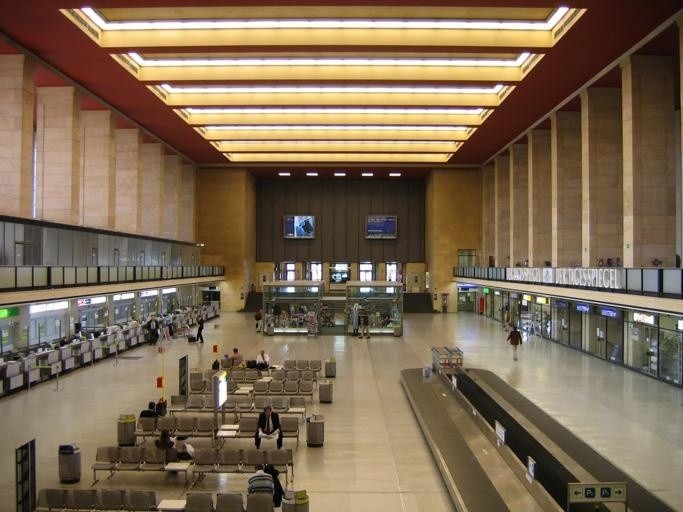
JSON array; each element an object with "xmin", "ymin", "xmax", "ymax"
[
  {"xmin": 306, "ymin": 414, "xmax": 324, "ymax": 447},
  {"xmin": 394, "ymin": 327, "xmax": 400, "ymax": 336},
  {"xmin": 325, "ymin": 357, "xmax": 336, "ymax": 378},
  {"xmin": 319, "ymin": 380, "xmax": 333, "ymax": 403},
  {"xmin": 267, "ymin": 323, "xmax": 273, "ymax": 335},
  {"xmin": 58, "ymin": 442, "xmax": 80, "ymax": 483},
  {"xmin": 282, "ymin": 489, "xmax": 309, "ymax": 512},
  {"xmin": 190, "ymin": 369, "xmax": 202, "ymax": 390},
  {"xmin": 116, "ymin": 414, "xmax": 134, "ymax": 446}
]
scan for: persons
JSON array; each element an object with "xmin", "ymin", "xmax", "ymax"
[
  {"xmin": 503, "ymin": 305, "xmax": 511, "ymax": 332},
  {"xmin": 154, "ymin": 429, "xmax": 176, "ymax": 448},
  {"xmin": 255, "ymin": 308, "xmax": 263, "ymax": 332},
  {"xmin": 223, "ymin": 347, "xmax": 244, "ymax": 361},
  {"xmin": 139, "ymin": 401, "xmax": 159, "ymax": 418},
  {"xmin": 254, "ymin": 406, "xmax": 283, "ymax": 449},
  {"xmin": 256, "ymin": 350, "xmax": 270, "ymax": 370},
  {"xmin": 146, "ymin": 312, "xmax": 173, "ymax": 345},
  {"xmin": 247, "ymin": 463, "xmax": 274, "ymax": 495},
  {"xmin": 196, "ymin": 315, "xmax": 204, "ymax": 343},
  {"xmin": 351, "ymin": 306, "xmax": 371, "ymax": 339},
  {"xmin": 595, "ymin": 255, "xmax": 613, "ymax": 267},
  {"xmin": 506, "ymin": 325, "xmax": 523, "ymax": 361}
]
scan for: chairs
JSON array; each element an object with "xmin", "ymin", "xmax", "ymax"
[
  {"xmin": 132, "ymin": 416, "xmax": 300, "ymax": 450},
  {"xmin": 35, "ymin": 488, "xmax": 276, "ymax": 512},
  {"xmin": 93, "ymin": 445, "xmax": 294, "ymax": 487},
  {"xmin": 169, "ymin": 356, "xmax": 323, "ymax": 421}
]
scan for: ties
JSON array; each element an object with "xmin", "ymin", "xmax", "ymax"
[{"xmin": 266, "ymin": 416, "xmax": 270, "ymax": 435}]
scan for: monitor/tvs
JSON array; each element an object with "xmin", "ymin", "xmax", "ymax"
[
  {"xmin": 365, "ymin": 214, "xmax": 397, "ymax": 240},
  {"xmin": 282, "ymin": 213, "xmax": 316, "ymax": 239}
]
